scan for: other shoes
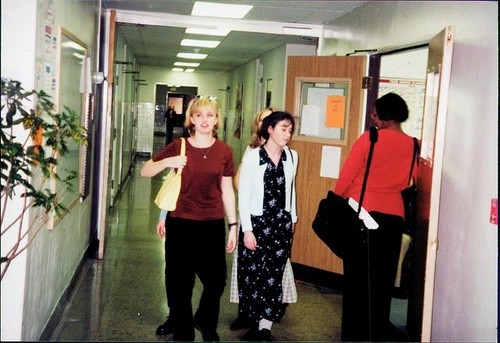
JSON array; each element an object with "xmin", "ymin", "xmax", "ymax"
[
  {"xmin": 229, "ymin": 314, "xmax": 249, "ymax": 330},
  {"xmin": 255, "ymin": 323, "xmax": 273, "ymax": 343},
  {"xmin": 198, "ymin": 328, "xmax": 220, "ymax": 343}
]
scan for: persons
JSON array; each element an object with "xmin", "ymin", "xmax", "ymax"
[
  {"xmin": 140, "ymin": 96, "xmax": 237, "ymax": 342},
  {"xmin": 332, "ymin": 92, "xmax": 418, "ymax": 343},
  {"xmin": 230, "ymin": 107, "xmax": 299, "ymax": 331},
  {"xmin": 238, "ymin": 111, "xmax": 298, "ymax": 343}
]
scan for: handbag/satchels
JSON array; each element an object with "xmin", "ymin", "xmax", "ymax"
[
  {"xmin": 154, "ymin": 135, "xmax": 186, "ymax": 212},
  {"xmin": 311, "ymin": 190, "xmax": 374, "ymax": 262}
]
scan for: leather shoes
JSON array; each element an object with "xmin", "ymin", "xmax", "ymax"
[{"xmin": 154, "ymin": 318, "xmax": 174, "ymax": 336}]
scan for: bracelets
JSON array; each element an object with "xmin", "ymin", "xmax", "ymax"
[{"xmin": 227, "ymin": 222, "xmax": 238, "ymax": 228}]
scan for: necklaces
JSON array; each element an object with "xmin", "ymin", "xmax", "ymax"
[{"xmin": 194, "ymin": 137, "xmax": 213, "ymax": 159}]
